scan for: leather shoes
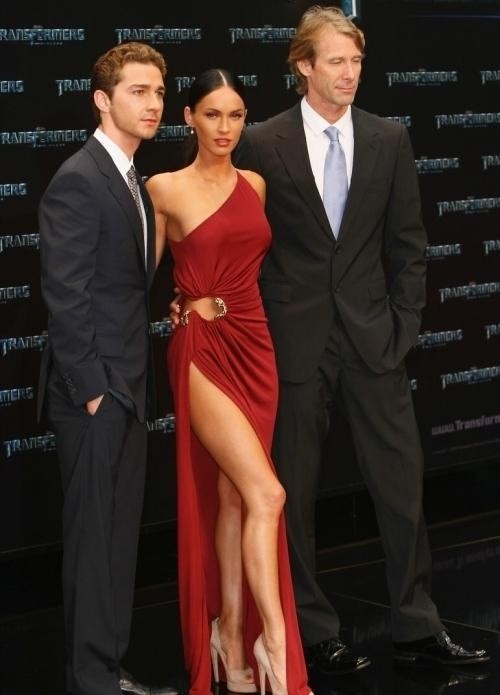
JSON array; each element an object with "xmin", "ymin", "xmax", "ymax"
[
  {"xmin": 390, "ymin": 632, "xmax": 490, "ymax": 667},
  {"xmin": 304, "ymin": 639, "xmax": 372, "ymax": 677},
  {"xmin": 119, "ymin": 669, "xmax": 178, "ymax": 695}
]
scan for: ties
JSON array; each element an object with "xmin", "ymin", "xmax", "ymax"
[
  {"xmin": 126, "ymin": 165, "xmax": 145, "ymax": 235},
  {"xmin": 323, "ymin": 127, "xmax": 350, "ymax": 238}
]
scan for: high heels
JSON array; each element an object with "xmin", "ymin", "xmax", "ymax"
[
  {"xmin": 210, "ymin": 617, "xmax": 256, "ymax": 692},
  {"xmin": 252, "ymin": 633, "xmax": 313, "ymax": 694}
]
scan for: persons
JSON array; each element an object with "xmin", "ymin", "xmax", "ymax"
[
  {"xmin": 142, "ymin": 66, "xmax": 318, "ymax": 695},
  {"xmin": 229, "ymin": 2, "xmax": 492, "ymax": 677},
  {"xmin": 35, "ymin": 42, "xmax": 171, "ymax": 695}
]
